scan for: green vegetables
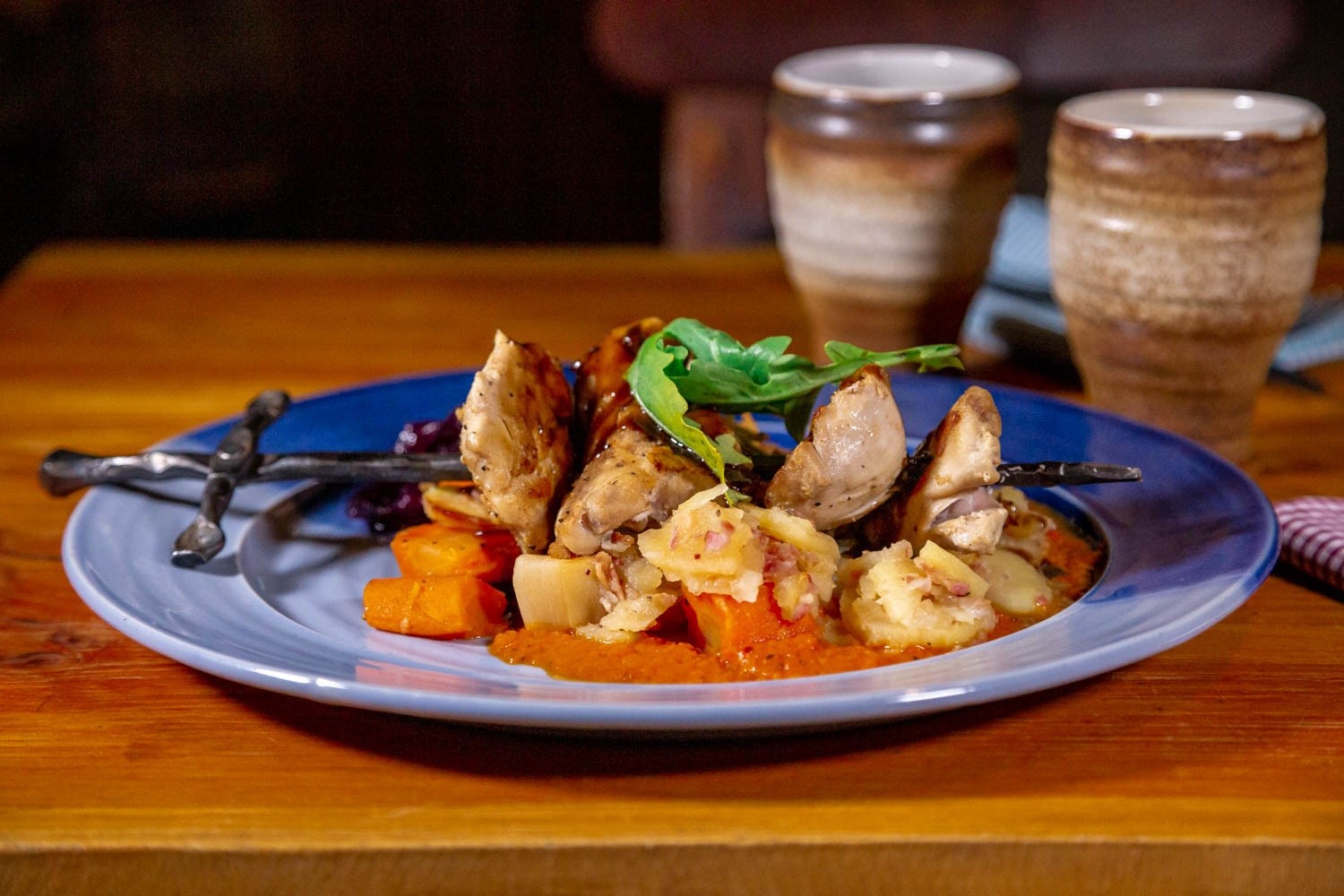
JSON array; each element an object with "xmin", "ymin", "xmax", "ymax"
[{"xmin": 622, "ymin": 316, "xmax": 962, "ymax": 500}]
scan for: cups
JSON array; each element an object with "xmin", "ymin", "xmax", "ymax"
[
  {"xmin": 764, "ymin": 44, "xmax": 1025, "ymax": 364},
  {"xmin": 1046, "ymin": 85, "xmax": 1327, "ymax": 463}
]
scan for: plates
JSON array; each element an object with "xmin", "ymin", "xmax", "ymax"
[{"xmin": 59, "ymin": 364, "xmax": 1285, "ymax": 730}]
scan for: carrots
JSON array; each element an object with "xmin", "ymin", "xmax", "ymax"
[{"xmin": 364, "ymin": 521, "xmax": 819, "ymax": 654}]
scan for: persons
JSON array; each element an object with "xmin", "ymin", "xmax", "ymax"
[{"xmin": 587, "ymin": 0, "xmax": 1344, "ymax": 251}]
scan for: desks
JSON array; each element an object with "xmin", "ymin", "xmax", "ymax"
[{"xmin": 0, "ymin": 239, "xmax": 1344, "ymax": 896}]
